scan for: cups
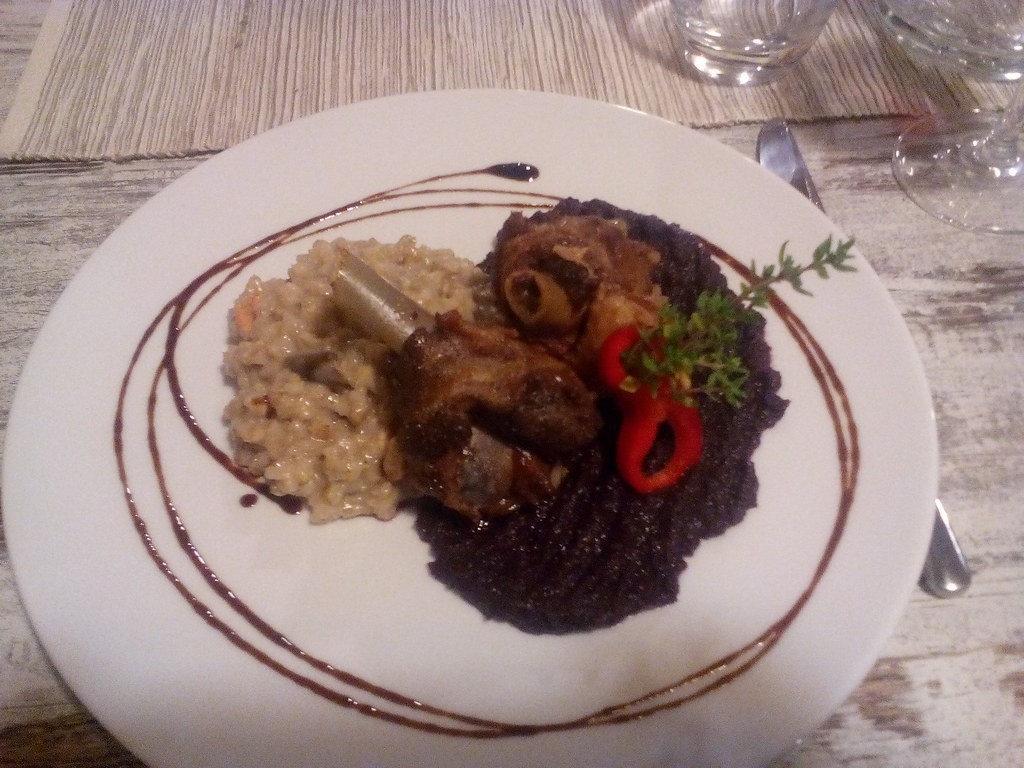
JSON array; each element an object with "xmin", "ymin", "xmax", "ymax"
[
  {"xmin": 669, "ymin": 0, "xmax": 837, "ymax": 89},
  {"xmin": 875, "ymin": 0, "xmax": 1024, "ymax": 82}
]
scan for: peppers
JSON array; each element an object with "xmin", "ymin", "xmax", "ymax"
[{"xmin": 602, "ymin": 321, "xmax": 703, "ymax": 492}]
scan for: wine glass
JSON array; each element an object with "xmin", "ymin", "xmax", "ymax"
[{"xmin": 890, "ymin": 68, "xmax": 1024, "ymax": 238}]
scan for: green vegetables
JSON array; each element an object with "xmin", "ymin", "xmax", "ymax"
[{"xmin": 622, "ymin": 234, "xmax": 858, "ymax": 411}]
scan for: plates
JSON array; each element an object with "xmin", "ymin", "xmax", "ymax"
[{"xmin": 0, "ymin": 86, "xmax": 942, "ymax": 768}]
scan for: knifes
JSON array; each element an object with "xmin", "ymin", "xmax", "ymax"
[{"xmin": 755, "ymin": 117, "xmax": 972, "ymax": 600}]
